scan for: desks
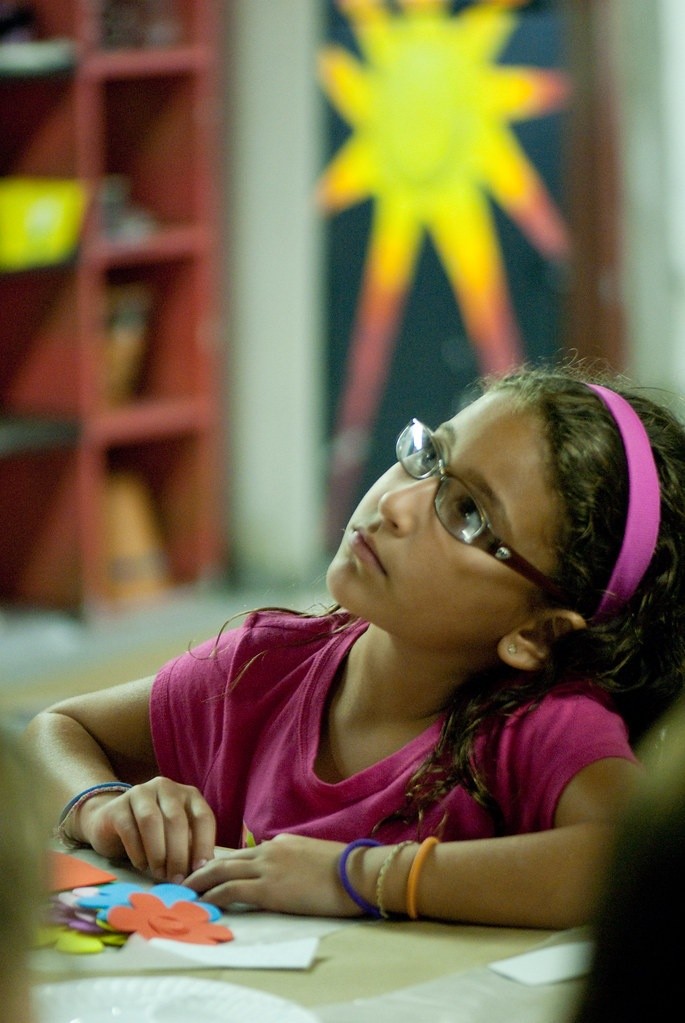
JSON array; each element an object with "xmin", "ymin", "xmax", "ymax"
[{"xmin": 24, "ymin": 832, "xmax": 591, "ymax": 1023}]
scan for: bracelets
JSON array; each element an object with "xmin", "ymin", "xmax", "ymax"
[
  {"xmin": 405, "ymin": 836, "xmax": 437, "ymax": 920},
  {"xmin": 51, "ymin": 782, "xmax": 133, "ymax": 852},
  {"xmin": 337, "ymin": 836, "xmax": 383, "ymax": 919},
  {"xmin": 374, "ymin": 840, "xmax": 413, "ymax": 920}
]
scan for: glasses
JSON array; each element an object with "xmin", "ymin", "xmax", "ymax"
[{"xmin": 394, "ymin": 417, "xmax": 569, "ymax": 602}]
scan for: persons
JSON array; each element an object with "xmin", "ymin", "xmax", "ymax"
[{"xmin": 20, "ymin": 369, "xmax": 685, "ymax": 930}]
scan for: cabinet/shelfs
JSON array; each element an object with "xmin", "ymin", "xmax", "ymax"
[{"xmin": 0, "ymin": 0, "xmax": 231, "ymax": 634}]
